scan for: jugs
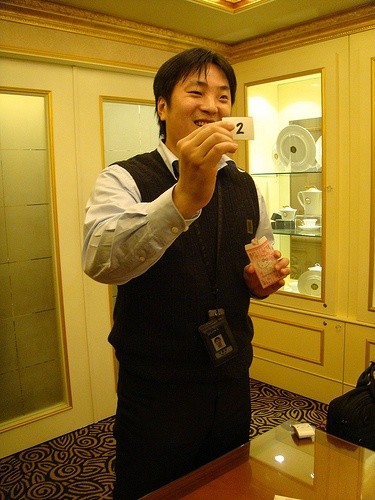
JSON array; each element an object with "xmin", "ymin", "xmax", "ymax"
[{"xmin": 296, "ymin": 188, "xmax": 322, "ymax": 216}]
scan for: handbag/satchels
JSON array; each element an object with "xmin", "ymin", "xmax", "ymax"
[{"xmin": 326, "ymin": 363, "xmax": 375, "ymax": 452}]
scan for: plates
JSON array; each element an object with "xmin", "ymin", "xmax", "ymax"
[
  {"xmin": 298, "ymin": 270, "xmax": 321, "ymax": 297},
  {"xmin": 276, "ymin": 124, "xmax": 316, "ymax": 172},
  {"xmin": 298, "ymin": 226, "xmax": 322, "ymax": 231}
]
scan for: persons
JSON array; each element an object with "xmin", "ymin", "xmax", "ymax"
[
  {"xmin": 214, "ymin": 337, "xmax": 226, "ymax": 351},
  {"xmin": 82, "ymin": 47, "xmax": 289, "ymax": 500}
]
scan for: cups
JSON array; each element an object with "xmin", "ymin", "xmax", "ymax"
[
  {"xmin": 280, "ymin": 207, "xmax": 297, "ymax": 221},
  {"xmin": 303, "ymin": 219, "xmax": 317, "ymax": 227}
]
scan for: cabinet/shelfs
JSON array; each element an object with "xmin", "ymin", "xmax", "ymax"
[{"xmin": 228, "ymin": 26, "xmax": 375, "ymax": 406}]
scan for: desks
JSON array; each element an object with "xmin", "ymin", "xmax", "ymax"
[{"xmin": 141, "ymin": 416, "xmax": 375, "ymax": 500}]
